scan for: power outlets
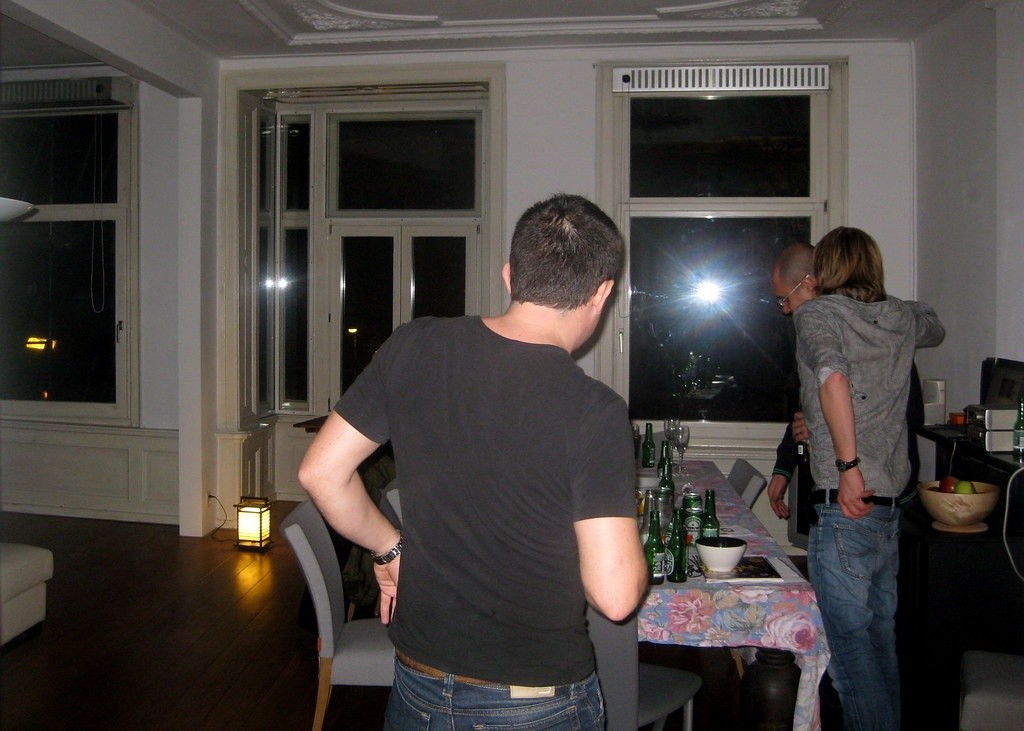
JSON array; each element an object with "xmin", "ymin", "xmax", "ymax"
[{"xmin": 206, "ymin": 490, "xmax": 212, "ymax": 507}]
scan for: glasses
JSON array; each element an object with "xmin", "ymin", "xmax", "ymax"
[{"xmin": 775, "ymin": 273, "xmax": 812, "ymax": 308}]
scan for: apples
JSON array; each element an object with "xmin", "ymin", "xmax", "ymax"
[{"xmin": 937, "ymin": 475, "xmax": 978, "ymax": 494}]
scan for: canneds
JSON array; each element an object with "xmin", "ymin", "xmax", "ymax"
[
  {"xmin": 653, "ymin": 486, "xmax": 673, "ymax": 528},
  {"xmin": 681, "ymin": 492, "xmax": 706, "ymax": 548}
]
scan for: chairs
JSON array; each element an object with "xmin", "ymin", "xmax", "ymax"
[
  {"xmin": 583, "ymin": 602, "xmax": 702, "ymax": 731},
  {"xmin": 279, "ymin": 497, "xmax": 396, "ymax": 731},
  {"xmin": 727, "ymin": 459, "xmax": 764, "ymax": 507}
]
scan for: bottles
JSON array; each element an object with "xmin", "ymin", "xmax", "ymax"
[
  {"xmin": 657, "ymin": 441, "xmax": 670, "ymax": 477},
  {"xmin": 666, "ymin": 508, "xmax": 688, "ymax": 582},
  {"xmin": 1012, "ymin": 399, "xmax": 1024, "ymax": 458},
  {"xmin": 659, "ymin": 458, "xmax": 674, "ymax": 497},
  {"xmin": 642, "ymin": 423, "xmax": 656, "ymax": 467},
  {"xmin": 644, "ymin": 511, "xmax": 664, "ymax": 585},
  {"xmin": 796, "ymin": 439, "xmax": 810, "ymax": 469},
  {"xmin": 639, "ymin": 489, "xmax": 662, "ymax": 547},
  {"xmin": 700, "ymin": 489, "xmax": 720, "ymax": 539}
]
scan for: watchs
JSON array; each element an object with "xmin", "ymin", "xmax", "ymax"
[{"xmin": 835, "ymin": 457, "xmax": 860, "ymax": 472}]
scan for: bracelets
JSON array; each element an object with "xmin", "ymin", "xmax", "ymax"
[{"xmin": 371, "ymin": 528, "xmax": 403, "ymax": 565}]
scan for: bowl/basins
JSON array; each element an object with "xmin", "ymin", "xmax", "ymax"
[
  {"xmin": 917, "ymin": 480, "xmax": 1001, "ymax": 528},
  {"xmin": 695, "ymin": 537, "xmax": 747, "ymax": 572}
]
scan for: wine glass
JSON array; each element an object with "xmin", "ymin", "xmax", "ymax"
[
  {"xmin": 663, "ymin": 416, "xmax": 680, "ymax": 458},
  {"xmin": 673, "ymin": 425, "xmax": 690, "ymax": 477}
]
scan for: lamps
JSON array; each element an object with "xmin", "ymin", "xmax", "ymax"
[
  {"xmin": 234, "ymin": 493, "xmax": 275, "ymax": 552},
  {"xmin": 0, "ymin": 196, "xmax": 35, "ymax": 223}
]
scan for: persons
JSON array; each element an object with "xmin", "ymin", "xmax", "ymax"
[
  {"xmin": 767, "ymin": 239, "xmax": 924, "ymax": 731},
  {"xmin": 793, "ymin": 226, "xmax": 946, "ymax": 731},
  {"xmin": 298, "ymin": 195, "xmax": 651, "ymax": 731}
]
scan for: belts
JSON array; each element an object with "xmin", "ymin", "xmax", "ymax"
[
  {"xmin": 805, "ymin": 489, "xmax": 900, "ymax": 524},
  {"xmin": 395, "ymin": 648, "xmax": 504, "ymax": 687}
]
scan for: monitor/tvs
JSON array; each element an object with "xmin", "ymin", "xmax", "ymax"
[{"xmin": 788, "ymin": 464, "xmax": 815, "ymax": 551}]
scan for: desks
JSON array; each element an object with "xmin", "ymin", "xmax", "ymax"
[
  {"xmin": 636, "ymin": 460, "xmax": 832, "ymax": 731},
  {"xmin": 915, "ymin": 422, "xmax": 1024, "ymax": 653}
]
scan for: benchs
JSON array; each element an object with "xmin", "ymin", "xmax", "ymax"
[{"xmin": 0, "ymin": 542, "xmax": 54, "ymax": 646}]
